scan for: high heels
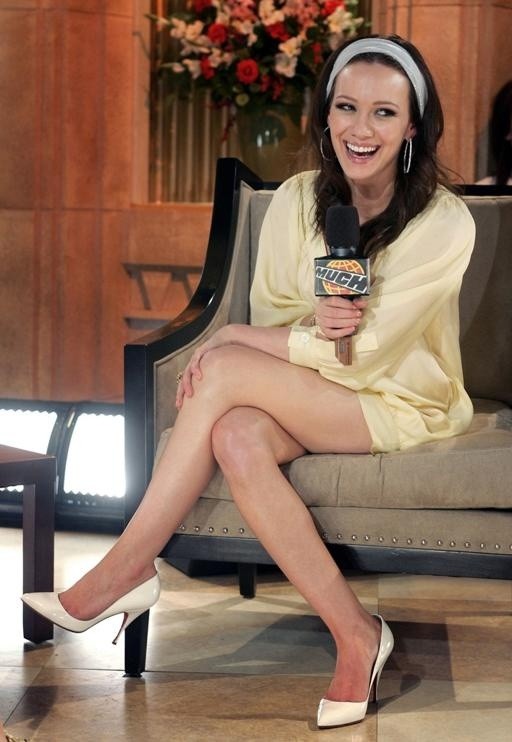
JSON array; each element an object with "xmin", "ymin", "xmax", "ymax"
[
  {"xmin": 22, "ymin": 566, "xmax": 162, "ymax": 646},
  {"xmin": 314, "ymin": 611, "xmax": 396, "ymax": 730}
]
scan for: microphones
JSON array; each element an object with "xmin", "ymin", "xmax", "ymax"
[{"xmin": 314, "ymin": 205, "xmax": 371, "ymax": 366}]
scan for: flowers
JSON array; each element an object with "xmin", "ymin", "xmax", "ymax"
[{"xmin": 132, "ymin": 1, "xmax": 373, "ymax": 146}]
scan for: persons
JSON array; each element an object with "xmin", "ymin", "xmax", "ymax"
[{"xmin": 19, "ymin": 35, "xmax": 475, "ymax": 731}]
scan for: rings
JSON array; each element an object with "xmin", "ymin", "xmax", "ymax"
[{"xmin": 175, "ymin": 371, "xmax": 183, "ymax": 383}]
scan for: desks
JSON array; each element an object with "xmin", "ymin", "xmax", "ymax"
[{"xmin": 0, "ymin": 442, "xmax": 56, "ymax": 644}]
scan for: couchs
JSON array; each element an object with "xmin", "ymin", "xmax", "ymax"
[{"xmin": 124, "ymin": 157, "xmax": 511, "ymax": 678}]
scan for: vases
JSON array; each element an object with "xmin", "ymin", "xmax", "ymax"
[{"xmin": 236, "ymin": 107, "xmax": 295, "ymax": 194}]
